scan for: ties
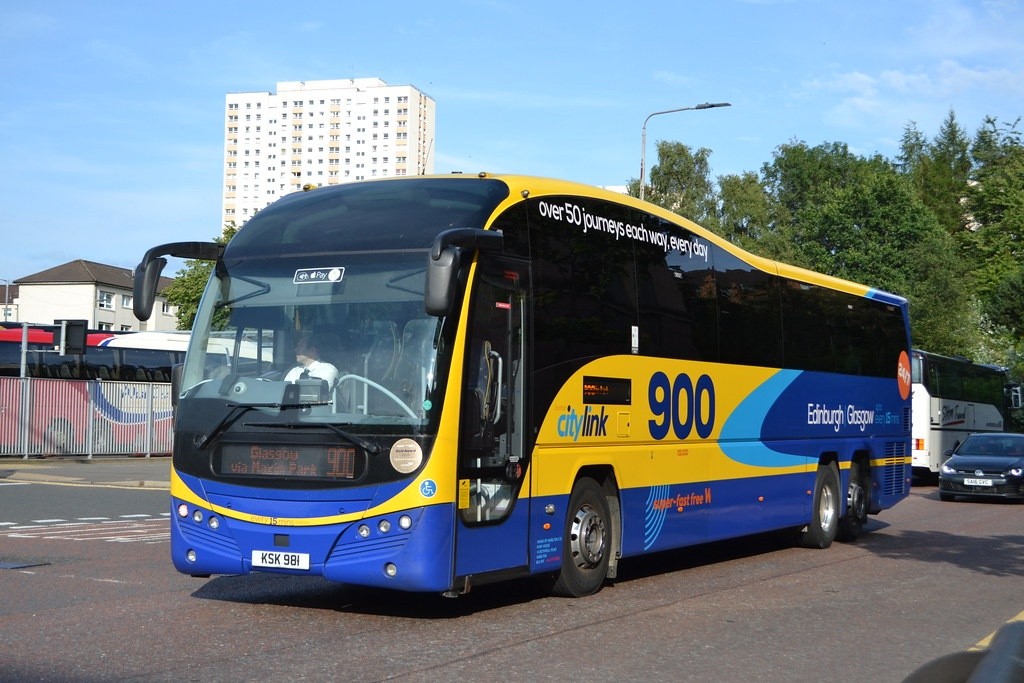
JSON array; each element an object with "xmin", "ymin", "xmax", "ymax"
[{"xmin": 297, "ymin": 369, "xmax": 310, "ymax": 384}]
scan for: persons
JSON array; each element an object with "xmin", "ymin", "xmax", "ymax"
[{"xmin": 283, "ymin": 336, "xmax": 338, "ymax": 413}]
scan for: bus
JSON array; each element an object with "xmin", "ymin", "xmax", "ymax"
[
  {"xmin": 132, "ymin": 173, "xmax": 914, "ymax": 597},
  {"xmin": 912, "ymin": 348, "xmax": 1011, "ymax": 473},
  {"xmin": 0, "ymin": 333, "xmax": 273, "ymax": 458}
]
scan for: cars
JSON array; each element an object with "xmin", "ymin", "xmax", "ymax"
[{"xmin": 940, "ymin": 432, "xmax": 1024, "ymax": 501}]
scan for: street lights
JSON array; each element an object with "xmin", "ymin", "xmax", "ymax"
[
  {"xmin": 0, "ymin": 279, "xmax": 10, "ymax": 320},
  {"xmin": 640, "ymin": 102, "xmax": 731, "ymax": 199}
]
